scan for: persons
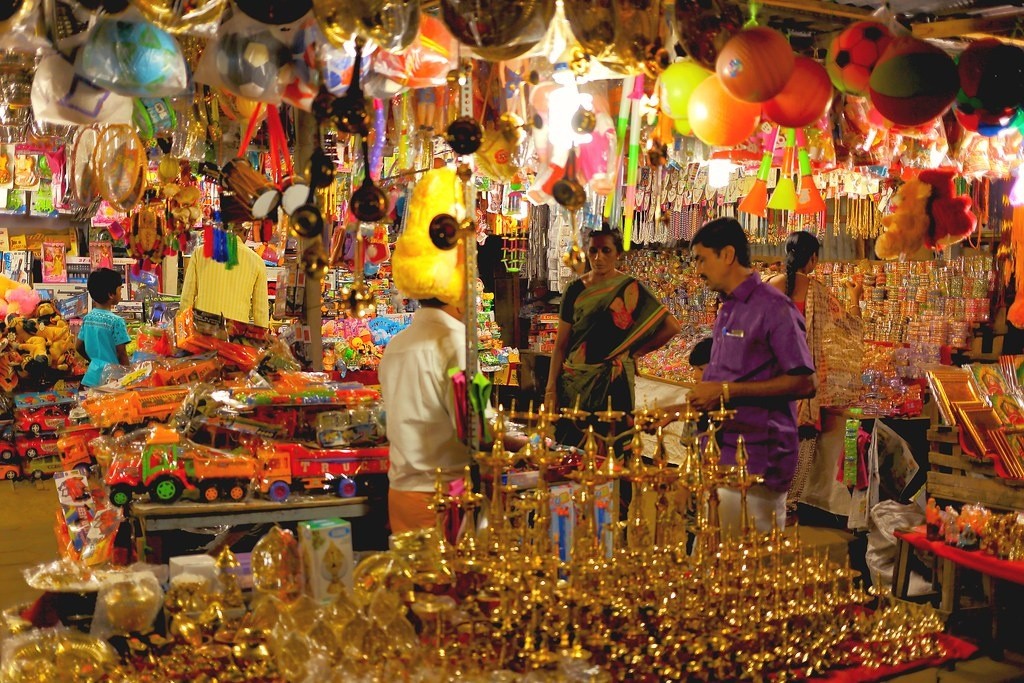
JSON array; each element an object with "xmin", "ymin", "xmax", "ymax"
[
  {"xmin": 635, "ymin": 216, "xmax": 818, "ymax": 541},
  {"xmin": 543, "ymin": 221, "xmax": 681, "ymax": 550},
  {"xmin": 379, "ymin": 297, "xmax": 530, "ymax": 539},
  {"xmin": 76, "ymin": 268, "xmax": 132, "ymax": 393},
  {"xmin": 759, "ymin": 231, "xmax": 866, "ymax": 526},
  {"xmin": 181, "ymin": 218, "xmax": 269, "ymax": 329}
]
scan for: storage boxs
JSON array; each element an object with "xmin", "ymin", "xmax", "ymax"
[{"xmin": 2, "ymin": 243, "xmax": 144, "ymax": 321}]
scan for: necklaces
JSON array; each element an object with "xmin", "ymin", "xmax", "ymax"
[
  {"xmin": 605, "ymin": 268, "xmax": 616, "ymax": 274},
  {"xmin": 795, "ymin": 272, "xmax": 808, "ymax": 277}
]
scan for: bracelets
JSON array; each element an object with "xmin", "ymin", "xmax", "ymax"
[
  {"xmin": 722, "ymin": 380, "xmax": 729, "ymax": 402},
  {"xmin": 848, "ymin": 305, "xmax": 862, "ymax": 313},
  {"xmin": 545, "ymin": 392, "xmax": 555, "ymax": 396}
]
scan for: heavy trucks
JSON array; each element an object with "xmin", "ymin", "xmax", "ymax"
[{"xmin": 54, "ymin": 357, "xmax": 391, "ymax": 508}]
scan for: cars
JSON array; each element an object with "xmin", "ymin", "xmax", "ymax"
[{"xmin": 0, "ymin": 406, "xmax": 76, "ymax": 482}]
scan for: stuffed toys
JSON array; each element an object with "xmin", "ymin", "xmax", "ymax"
[
  {"xmin": 0, "ymin": 275, "xmax": 75, "ymax": 385},
  {"xmin": 876, "ymin": 162, "xmax": 976, "ymax": 263}
]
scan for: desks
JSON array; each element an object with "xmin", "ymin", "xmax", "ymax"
[
  {"xmin": 131, "ymin": 485, "xmax": 368, "ymax": 563},
  {"xmin": 634, "ymin": 373, "xmax": 931, "ymax": 528},
  {"xmin": 891, "ymin": 531, "xmax": 1024, "ymax": 672}
]
